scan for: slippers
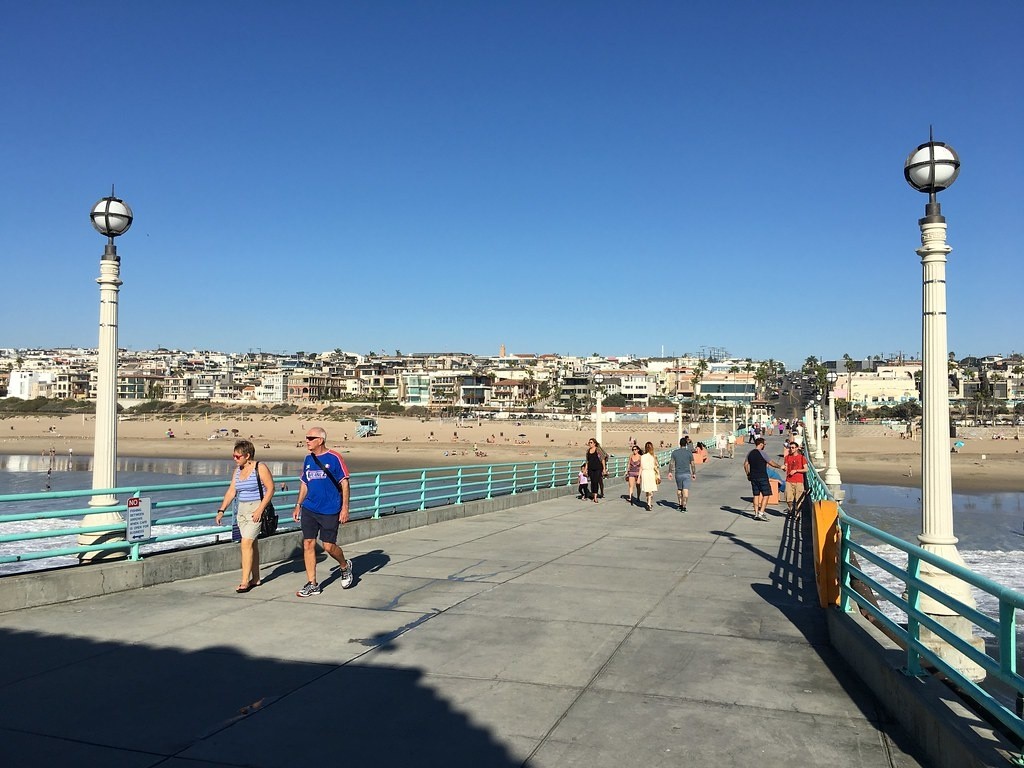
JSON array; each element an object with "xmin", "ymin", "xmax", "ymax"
[
  {"xmin": 235, "ymin": 584, "xmax": 251, "ymax": 593},
  {"xmin": 249, "ymin": 579, "xmax": 262, "ymax": 587}
]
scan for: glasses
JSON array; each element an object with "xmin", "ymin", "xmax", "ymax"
[
  {"xmin": 632, "ymin": 449, "xmax": 637, "ymax": 451},
  {"xmin": 233, "ymin": 454, "xmax": 247, "ymax": 460},
  {"xmin": 306, "ymin": 436, "xmax": 324, "ymax": 441},
  {"xmin": 789, "ymin": 447, "xmax": 797, "ymax": 449}
]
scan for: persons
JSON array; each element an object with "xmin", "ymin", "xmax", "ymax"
[
  {"xmin": 42, "ymin": 424, "xmax": 72, "ymax": 491},
  {"xmin": 214, "ymin": 438, "xmax": 289, "ymax": 594},
  {"xmin": 748, "ymin": 416, "xmax": 804, "ymax": 454},
  {"xmin": 783, "ymin": 442, "xmax": 809, "ymax": 513},
  {"xmin": 743, "ymin": 438, "xmax": 786, "ymax": 522},
  {"xmin": 292, "ymin": 427, "xmax": 355, "ymax": 598},
  {"xmin": 577, "ymin": 438, "xmax": 609, "ymax": 503},
  {"xmin": 908, "ymin": 464, "xmax": 913, "ymax": 478},
  {"xmin": 623, "ymin": 445, "xmax": 644, "ymax": 502},
  {"xmin": 451, "ymin": 443, "xmax": 487, "ymax": 457},
  {"xmin": 718, "ymin": 431, "xmax": 736, "ymax": 459},
  {"xmin": 685, "ymin": 435, "xmax": 710, "ymax": 465},
  {"xmin": 637, "ymin": 441, "xmax": 659, "ymax": 511},
  {"xmin": 668, "ymin": 438, "xmax": 695, "ymax": 512}
]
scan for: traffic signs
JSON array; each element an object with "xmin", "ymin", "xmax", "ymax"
[{"xmin": 126, "ymin": 497, "xmax": 151, "ymax": 543}]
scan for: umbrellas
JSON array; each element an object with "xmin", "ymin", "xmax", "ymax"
[{"xmin": 954, "ymin": 440, "xmax": 965, "ymax": 450}]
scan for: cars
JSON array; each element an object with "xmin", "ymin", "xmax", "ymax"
[
  {"xmin": 456, "ymin": 412, "xmax": 496, "ymax": 419},
  {"xmin": 508, "ymin": 414, "xmax": 559, "ymax": 420},
  {"xmin": 765, "ymin": 404, "xmax": 775, "ymax": 412},
  {"xmin": 770, "ymin": 372, "xmax": 820, "ymax": 400}
]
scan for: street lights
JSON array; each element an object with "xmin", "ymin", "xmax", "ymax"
[
  {"xmin": 814, "ymin": 393, "xmax": 825, "ymax": 462},
  {"xmin": 732, "ymin": 401, "xmax": 736, "ymax": 432},
  {"xmin": 903, "ymin": 125, "xmax": 989, "ymax": 686},
  {"xmin": 824, "ymin": 370, "xmax": 842, "ymax": 490},
  {"xmin": 677, "ymin": 393, "xmax": 684, "ymax": 449},
  {"xmin": 804, "ymin": 400, "xmax": 816, "ymax": 459},
  {"xmin": 592, "ymin": 372, "xmax": 604, "ymax": 449},
  {"xmin": 713, "ymin": 398, "xmax": 717, "ymax": 437},
  {"xmin": 78, "ymin": 182, "xmax": 135, "ymax": 565}
]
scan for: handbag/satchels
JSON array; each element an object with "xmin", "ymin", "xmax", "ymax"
[
  {"xmin": 261, "ymin": 502, "xmax": 278, "ymax": 537},
  {"xmin": 656, "ymin": 477, "xmax": 661, "ymax": 485},
  {"xmin": 625, "ymin": 474, "xmax": 628, "ymax": 481}
]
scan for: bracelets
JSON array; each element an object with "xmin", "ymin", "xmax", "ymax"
[
  {"xmin": 295, "ymin": 503, "xmax": 301, "ymax": 507},
  {"xmin": 796, "ymin": 469, "xmax": 799, "ymax": 473},
  {"xmin": 217, "ymin": 510, "xmax": 225, "ymax": 513}
]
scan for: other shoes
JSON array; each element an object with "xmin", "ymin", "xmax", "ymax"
[
  {"xmin": 594, "ymin": 499, "xmax": 598, "ymax": 503},
  {"xmin": 582, "ymin": 495, "xmax": 587, "ymax": 501},
  {"xmin": 601, "ymin": 492, "xmax": 605, "ymax": 498},
  {"xmin": 627, "ymin": 498, "xmax": 632, "ymax": 503},
  {"xmin": 645, "ymin": 505, "xmax": 652, "ymax": 511},
  {"xmin": 635, "ymin": 498, "xmax": 641, "ymax": 503},
  {"xmin": 758, "ymin": 512, "xmax": 768, "ymax": 521},
  {"xmin": 795, "ymin": 514, "xmax": 800, "ymax": 521},
  {"xmin": 754, "ymin": 513, "xmax": 759, "ymax": 520},
  {"xmin": 783, "ymin": 507, "xmax": 793, "ymax": 513},
  {"xmin": 677, "ymin": 505, "xmax": 683, "ymax": 511},
  {"xmin": 682, "ymin": 508, "xmax": 688, "ymax": 513}
]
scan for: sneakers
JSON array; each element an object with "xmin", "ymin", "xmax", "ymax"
[
  {"xmin": 296, "ymin": 581, "xmax": 320, "ymax": 597},
  {"xmin": 339, "ymin": 558, "xmax": 353, "ymax": 589}
]
